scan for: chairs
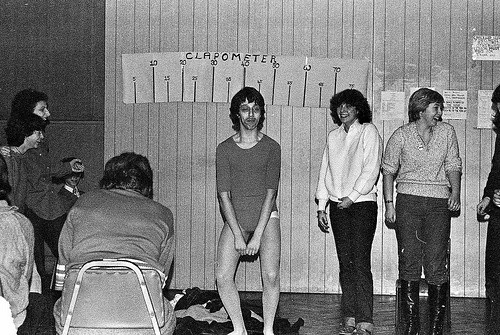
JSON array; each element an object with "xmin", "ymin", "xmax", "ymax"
[
  {"xmin": 396, "ymin": 238, "xmax": 450, "ymax": 332},
  {"xmin": 60, "ymin": 261, "xmax": 165, "ymax": 335}
]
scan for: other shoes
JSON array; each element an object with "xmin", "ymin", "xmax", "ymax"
[
  {"xmin": 339, "ymin": 324, "xmax": 355, "ymax": 335},
  {"xmin": 353, "ymin": 327, "xmax": 372, "ymax": 335}
]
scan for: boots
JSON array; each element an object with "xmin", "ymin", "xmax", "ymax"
[
  {"xmin": 484, "ymin": 295, "xmax": 496, "ymax": 335},
  {"xmin": 400, "ymin": 280, "xmax": 421, "ymax": 335},
  {"xmin": 425, "ymin": 281, "xmax": 449, "ymax": 335}
]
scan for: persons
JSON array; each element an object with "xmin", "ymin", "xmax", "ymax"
[
  {"xmin": 0, "ymin": 90, "xmax": 88, "ymax": 335},
  {"xmin": 52, "ymin": 153, "xmax": 177, "ymax": 335},
  {"xmin": 381, "ymin": 88, "xmax": 464, "ymax": 335},
  {"xmin": 315, "ymin": 89, "xmax": 383, "ymax": 335},
  {"xmin": 216, "ymin": 87, "xmax": 283, "ymax": 335},
  {"xmin": 477, "ymin": 86, "xmax": 500, "ymax": 335}
]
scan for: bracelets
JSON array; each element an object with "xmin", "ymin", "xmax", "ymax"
[
  {"xmin": 317, "ymin": 211, "xmax": 328, "ymax": 214},
  {"xmin": 384, "ymin": 200, "xmax": 393, "ymax": 203}
]
